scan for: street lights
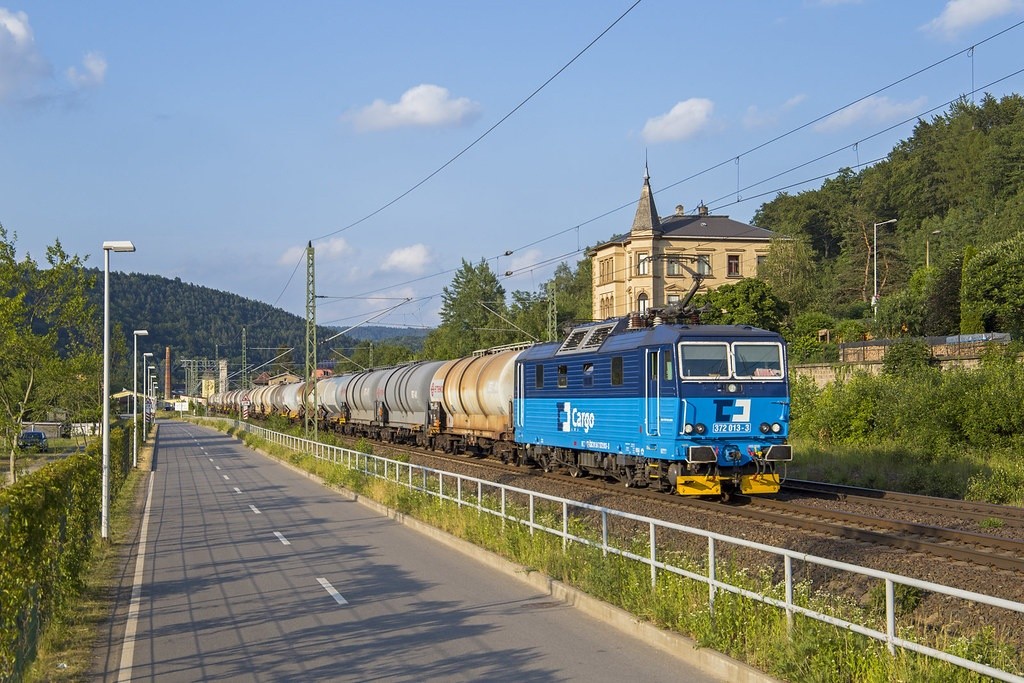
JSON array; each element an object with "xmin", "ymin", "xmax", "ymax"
[
  {"xmin": 872, "ymin": 217, "xmax": 899, "ymax": 322},
  {"xmin": 96, "ymin": 238, "xmax": 136, "ymax": 540},
  {"xmin": 130, "ymin": 329, "xmax": 149, "ymax": 468},
  {"xmin": 925, "ymin": 230, "xmax": 943, "ymax": 269},
  {"xmin": 147, "ymin": 366, "xmax": 159, "ymax": 398},
  {"xmin": 142, "ymin": 352, "xmax": 154, "ymax": 443}
]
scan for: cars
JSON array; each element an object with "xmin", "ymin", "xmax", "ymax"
[{"xmin": 18, "ymin": 431, "xmax": 49, "ymax": 454}]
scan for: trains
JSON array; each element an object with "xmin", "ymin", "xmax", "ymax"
[{"xmin": 206, "ymin": 321, "xmax": 794, "ymax": 506}]
